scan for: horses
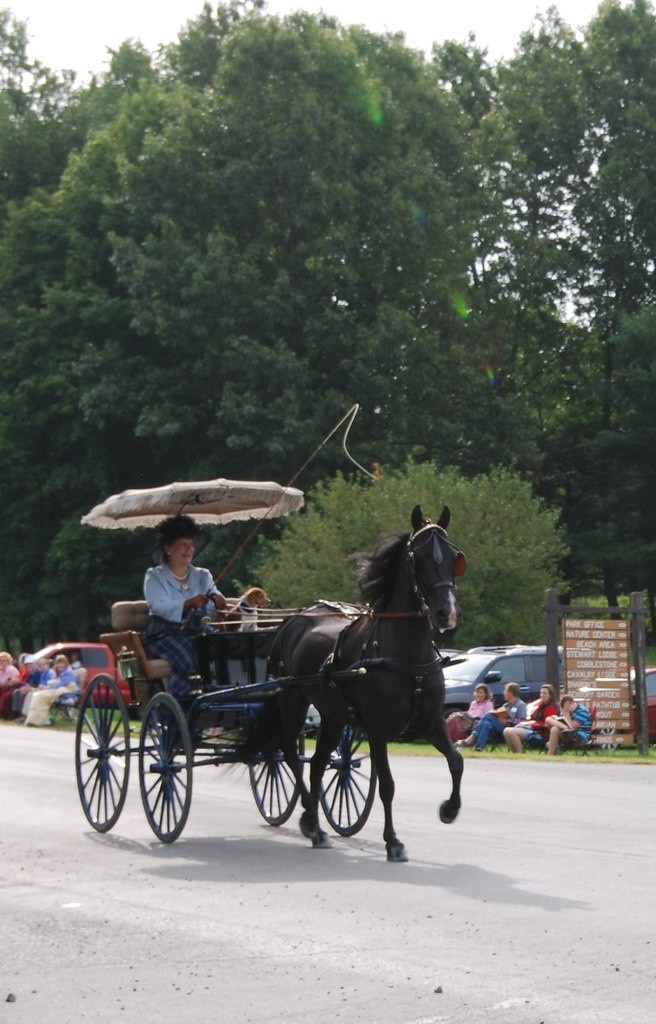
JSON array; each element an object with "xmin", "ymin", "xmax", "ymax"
[{"xmin": 266, "ymin": 504, "xmax": 467, "ymax": 862}]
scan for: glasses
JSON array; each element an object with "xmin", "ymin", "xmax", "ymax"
[{"xmin": 1, "ymin": 659, "xmax": 8, "ymax": 661}]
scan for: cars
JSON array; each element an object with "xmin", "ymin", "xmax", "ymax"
[
  {"xmin": 304, "ymin": 647, "xmax": 463, "ymax": 740},
  {"xmin": 528, "ymin": 665, "xmax": 656, "ymax": 738}
]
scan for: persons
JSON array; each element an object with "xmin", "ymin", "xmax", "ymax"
[
  {"xmin": 447, "ymin": 682, "xmax": 593, "ymax": 756},
  {"xmin": 143, "ymin": 517, "xmax": 271, "ymax": 696},
  {"xmin": 0, "ymin": 651, "xmax": 81, "ymax": 726}
]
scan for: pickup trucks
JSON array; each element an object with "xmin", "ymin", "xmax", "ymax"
[{"xmin": 17, "ymin": 641, "xmax": 138, "ymax": 707}]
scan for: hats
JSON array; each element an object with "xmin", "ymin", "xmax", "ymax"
[
  {"xmin": 151, "ymin": 516, "xmax": 210, "ymax": 564},
  {"xmin": 24, "ymin": 655, "xmax": 35, "ymax": 664}
]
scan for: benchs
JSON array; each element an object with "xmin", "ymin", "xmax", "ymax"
[{"xmin": 102, "ymin": 592, "xmax": 234, "ymax": 679}]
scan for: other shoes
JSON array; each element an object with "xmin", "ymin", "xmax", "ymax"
[
  {"xmin": 475, "ymin": 748, "xmax": 481, "ymax": 751},
  {"xmin": 456, "ymin": 739, "xmax": 472, "ymax": 747},
  {"xmin": 15, "ymin": 717, "xmax": 25, "ymax": 722}
]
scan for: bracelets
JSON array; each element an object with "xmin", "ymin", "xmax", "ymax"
[{"xmin": 188, "ymin": 599, "xmax": 191, "ymax": 609}]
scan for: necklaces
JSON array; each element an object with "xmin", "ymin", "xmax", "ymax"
[{"xmin": 167, "ymin": 565, "xmax": 189, "ymax": 590}]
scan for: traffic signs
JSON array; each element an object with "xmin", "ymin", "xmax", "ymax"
[{"xmin": 561, "ymin": 618, "xmax": 635, "ymax": 747}]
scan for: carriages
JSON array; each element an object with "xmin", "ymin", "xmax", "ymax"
[{"xmin": 74, "ymin": 505, "xmax": 468, "ymax": 862}]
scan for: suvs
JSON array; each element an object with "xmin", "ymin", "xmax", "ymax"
[{"xmin": 443, "ymin": 644, "xmax": 565, "ymax": 725}]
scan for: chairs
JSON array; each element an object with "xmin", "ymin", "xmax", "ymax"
[
  {"xmin": 52, "ymin": 667, "xmax": 88, "ymax": 724},
  {"xmin": 467, "ymin": 704, "xmax": 601, "ymax": 757}
]
